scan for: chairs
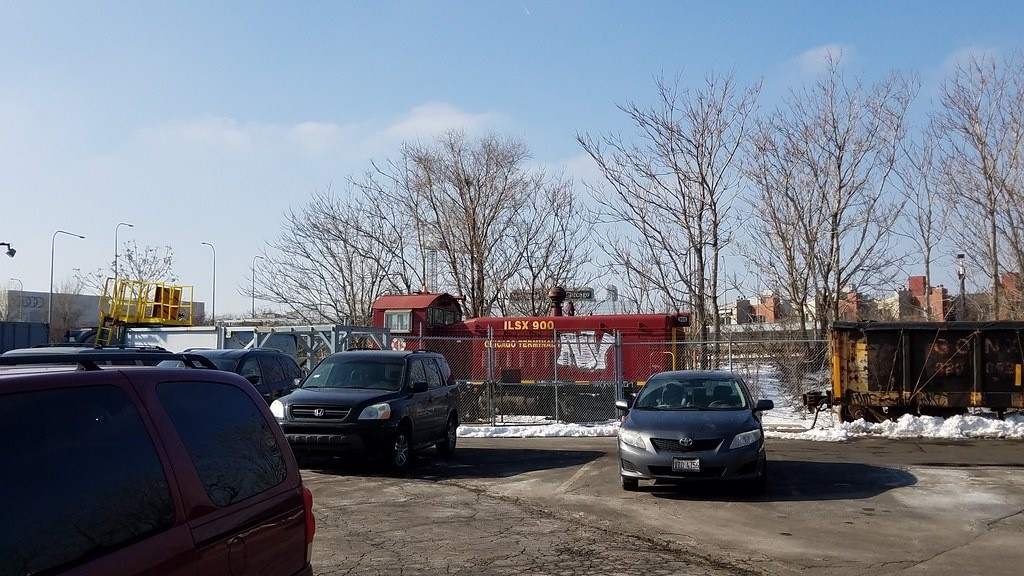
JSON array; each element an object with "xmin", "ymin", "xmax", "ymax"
[
  {"xmin": 662, "ymin": 383, "xmax": 682, "ymax": 403},
  {"xmin": 376, "ymin": 365, "xmax": 406, "ymax": 388},
  {"xmin": 348, "ymin": 365, "xmax": 366, "ymax": 388},
  {"xmin": 709, "ymin": 386, "xmax": 734, "ymax": 405}
]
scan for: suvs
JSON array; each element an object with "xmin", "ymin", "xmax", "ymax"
[
  {"xmin": 270, "ymin": 348, "xmax": 462, "ymax": 476},
  {"xmin": 0, "ymin": 354, "xmax": 316, "ymax": 576},
  {"xmin": 0, "ymin": 345, "xmax": 306, "ymax": 409}
]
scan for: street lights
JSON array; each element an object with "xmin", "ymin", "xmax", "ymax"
[
  {"xmin": 48, "ymin": 230, "xmax": 85, "ymax": 342},
  {"xmin": 115, "ymin": 222, "xmax": 134, "ymax": 297},
  {"xmin": 721, "ymin": 255, "xmax": 726, "ymax": 325},
  {"xmin": 10, "ymin": 277, "xmax": 23, "ymax": 322},
  {"xmin": 201, "ymin": 242, "xmax": 214, "ymax": 326},
  {"xmin": 251, "ymin": 255, "xmax": 264, "ymax": 318}
]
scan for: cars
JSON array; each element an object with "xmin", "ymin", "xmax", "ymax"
[{"xmin": 616, "ymin": 370, "xmax": 774, "ymax": 490}]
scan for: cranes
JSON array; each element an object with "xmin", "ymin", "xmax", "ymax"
[{"xmin": 388, "ymin": 238, "xmax": 476, "ymax": 294}]
